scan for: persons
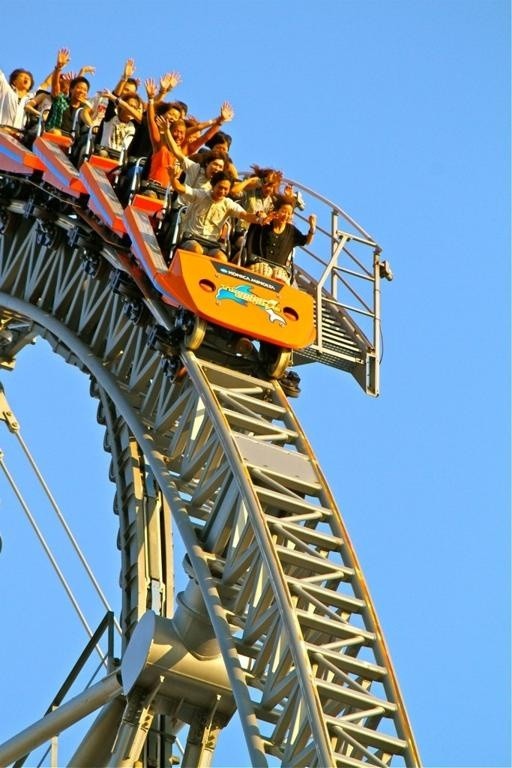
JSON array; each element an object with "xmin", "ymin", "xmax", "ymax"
[{"xmin": 1, "ymin": 50, "xmax": 317, "ymax": 284}]
